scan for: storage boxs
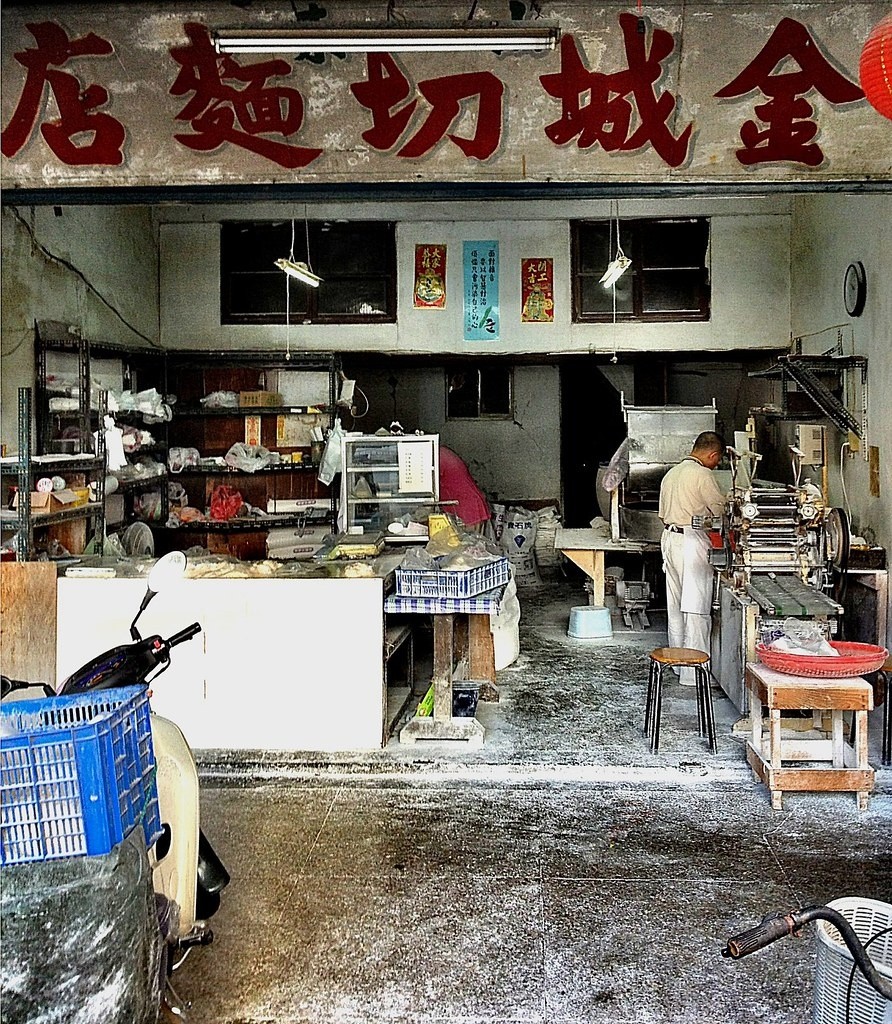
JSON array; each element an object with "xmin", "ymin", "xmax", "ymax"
[
  {"xmin": 0, "ymin": 684, "xmax": 166, "ymax": 868},
  {"xmin": 12, "ymin": 490, "xmax": 78, "ymax": 515},
  {"xmin": 396, "ymin": 558, "xmax": 510, "ymax": 600}
]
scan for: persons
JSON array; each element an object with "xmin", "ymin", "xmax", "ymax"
[
  {"xmin": 658, "ymin": 431, "xmax": 729, "ymax": 688},
  {"xmin": 439, "ymin": 445, "xmax": 492, "ymax": 536}
]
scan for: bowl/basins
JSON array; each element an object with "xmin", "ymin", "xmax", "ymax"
[{"xmin": 568, "ymin": 606, "xmax": 613, "ymax": 641}]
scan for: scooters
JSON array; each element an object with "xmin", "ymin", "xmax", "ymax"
[{"xmin": 0, "ymin": 552, "xmax": 230, "ymax": 1024}]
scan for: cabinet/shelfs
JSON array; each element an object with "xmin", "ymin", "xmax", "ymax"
[
  {"xmin": 47, "ymin": 389, "xmax": 334, "ymax": 551},
  {"xmin": 0, "ymin": 449, "xmax": 100, "ymax": 565}
]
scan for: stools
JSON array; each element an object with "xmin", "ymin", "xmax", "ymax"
[
  {"xmin": 644, "ymin": 646, "xmax": 717, "ymax": 757},
  {"xmin": 744, "ymin": 663, "xmax": 877, "ymax": 810}
]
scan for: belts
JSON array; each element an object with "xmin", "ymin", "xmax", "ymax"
[{"xmin": 665, "ymin": 524, "xmax": 685, "ymax": 533}]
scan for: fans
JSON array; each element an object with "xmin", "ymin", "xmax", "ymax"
[{"xmin": 122, "ymin": 522, "xmax": 155, "ymax": 560}]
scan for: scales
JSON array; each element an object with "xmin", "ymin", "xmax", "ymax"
[{"xmin": 326, "ymin": 529, "xmax": 386, "ymax": 561}]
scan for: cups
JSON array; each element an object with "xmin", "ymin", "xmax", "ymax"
[{"xmin": 310, "ymin": 441, "xmax": 325, "ymax": 465}]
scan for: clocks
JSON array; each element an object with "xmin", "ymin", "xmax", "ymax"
[{"xmin": 843, "ymin": 262, "xmax": 867, "ymax": 317}]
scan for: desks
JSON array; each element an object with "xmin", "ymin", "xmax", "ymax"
[
  {"xmin": 553, "ymin": 526, "xmax": 662, "ymax": 607},
  {"xmin": 384, "ymin": 585, "xmax": 512, "ymax": 744}
]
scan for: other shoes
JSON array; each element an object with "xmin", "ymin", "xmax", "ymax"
[{"xmin": 704, "ymin": 678, "xmax": 719, "ymax": 687}]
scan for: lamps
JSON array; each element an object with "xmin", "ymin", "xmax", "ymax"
[
  {"xmin": 598, "ymin": 199, "xmax": 632, "ymax": 289},
  {"xmin": 273, "ymin": 203, "xmax": 324, "ymax": 288}
]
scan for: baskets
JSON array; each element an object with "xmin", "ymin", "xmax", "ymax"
[
  {"xmin": 394, "ymin": 557, "xmax": 509, "ymax": 600},
  {"xmin": 811, "ymin": 896, "xmax": 892, "ymax": 1024},
  {"xmin": 755, "ymin": 636, "xmax": 889, "ymax": 677}
]
